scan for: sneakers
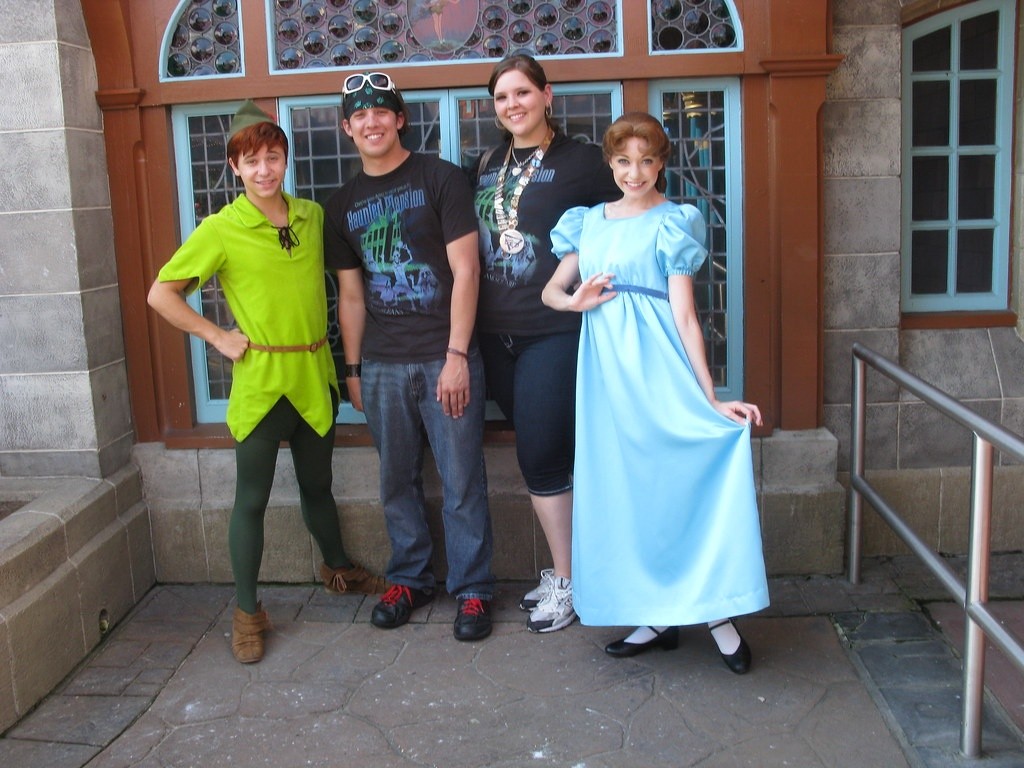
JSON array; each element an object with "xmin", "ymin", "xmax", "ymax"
[
  {"xmin": 453, "ymin": 599, "xmax": 493, "ymax": 642},
  {"xmin": 321, "ymin": 560, "xmax": 391, "ymax": 595},
  {"xmin": 371, "ymin": 584, "xmax": 435, "ymax": 630},
  {"xmin": 232, "ymin": 600, "xmax": 271, "ymax": 663},
  {"xmin": 519, "ymin": 567, "xmax": 577, "ymax": 633}
]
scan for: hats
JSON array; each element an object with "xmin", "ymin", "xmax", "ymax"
[{"xmin": 228, "ymin": 99, "xmax": 275, "ymax": 144}]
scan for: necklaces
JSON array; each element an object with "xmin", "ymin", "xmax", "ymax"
[{"xmin": 512, "ymin": 131, "xmax": 555, "ymax": 176}]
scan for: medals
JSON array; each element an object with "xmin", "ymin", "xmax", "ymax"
[{"xmin": 499, "ymin": 229, "xmax": 524, "ymax": 254}]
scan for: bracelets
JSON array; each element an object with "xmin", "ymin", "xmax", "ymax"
[
  {"xmin": 446, "ymin": 348, "xmax": 469, "ymax": 360},
  {"xmin": 344, "ymin": 364, "xmax": 361, "ymax": 377}
]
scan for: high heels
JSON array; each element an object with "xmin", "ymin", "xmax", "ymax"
[
  {"xmin": 708, "ymin": 618, "xmax": 752, "ymax": 674},
  {"xmin": 605, "ymin": 626, "xmax": 679, "ymax": 658}
]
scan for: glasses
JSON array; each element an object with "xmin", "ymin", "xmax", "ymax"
[{"xmin": 342, "ymin": 72, "xmax": 396, "ymax": 93}]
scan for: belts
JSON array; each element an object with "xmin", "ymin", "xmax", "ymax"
[{"xmin": 248, "ymin": 337, "xmax": 329, "ymax": 354}]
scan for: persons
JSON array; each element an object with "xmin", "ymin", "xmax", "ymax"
[
  {"xmin": 467, "ymin": 54, "xmax": 624, "ymax": 633},
  {"xmin": 540, "ymin": 111, "xmax": 771, "ymax": 674},
  {"xmin": 323, "ymin": 71, "xmax": 498, "ymax": 642},
  {"xmin": 145, "ymin": 99, "xmax": 381, "ymax": 664}
]
